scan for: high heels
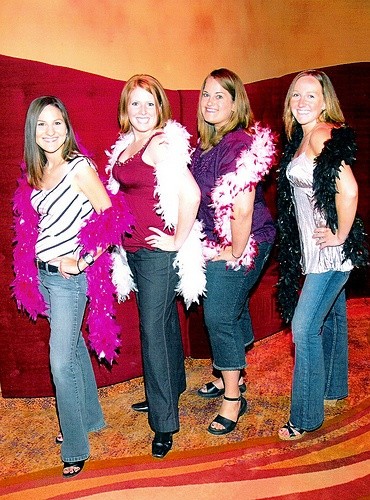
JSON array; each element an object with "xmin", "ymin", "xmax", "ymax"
[{"xmin": 278, "ymin": 419, "xmax": 307, "ymax": 440}]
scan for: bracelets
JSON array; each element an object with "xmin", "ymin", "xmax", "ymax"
[
  {"xmin": 232, "ymin": 253, "xmax": 242, "ymax": 259},
  {"xmin": 77, "ymin": 256, "xmax": 82, "ymax": 273}
]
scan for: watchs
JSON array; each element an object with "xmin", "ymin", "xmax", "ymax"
[{"xmin": 83, "ymin": 253, "xmax": 94, "ymax": 267}]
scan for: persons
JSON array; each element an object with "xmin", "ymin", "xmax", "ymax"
[
  {"xmin": 10, "ymin": 95, "xmax": 135, "ymax": 479},
  {"xmin": 187, "ymin": 68, "xmax": 277, "ymax": 435},
  {"xmin": 272, "ymin": 68, "xmax": 370, "ymax": 440},
  {"xmin": 104, "ymin": 73, "xmax": 208, "ymax": 459}
]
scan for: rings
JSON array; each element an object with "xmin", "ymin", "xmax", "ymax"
[{"xmin": 218, "ymin": 252, "xmax": 220, "ymax": 256}]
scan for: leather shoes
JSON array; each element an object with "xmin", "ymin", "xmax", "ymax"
[
  {"xmin": 151, "ymin": 431, "xmax": 173, "ymax": 458},
  {"xmin": 132, "ymin": 399, "xmax": 149, "ymax": 412}
]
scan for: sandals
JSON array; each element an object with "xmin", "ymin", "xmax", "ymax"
[
  {"xmin": 62, "ymin": 459, "xmax": 85, "ymax": 479},
  {"xmin": 56, "ymin": 432, "xmax": 63, "ymax": 443},
  {"xmin": 197, "ymin": 381, "xmax": 246, "ymax": 397},
  {"xmin": 208, "ymin": 395, "xmax": 247, "ymax": 435}
]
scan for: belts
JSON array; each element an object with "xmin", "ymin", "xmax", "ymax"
[{"xmin": 34, "ymin": 258, "xmax": 58, "ymax": 273}]
